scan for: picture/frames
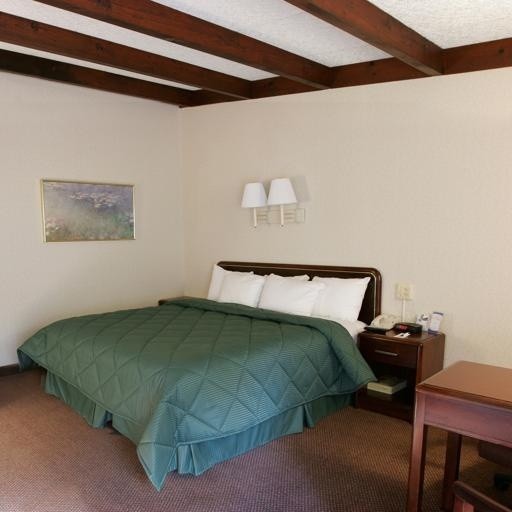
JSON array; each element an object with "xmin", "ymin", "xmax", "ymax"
[{"xmin": 40, "ymin": 179, "xmax": 138, "ymax": 243}]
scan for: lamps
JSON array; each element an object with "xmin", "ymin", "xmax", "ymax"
[
  {"xmin": 239, "ymin": 183, "xmax": 270, "ymax": 228},
  {"xmin": 266, "ymin": 178, "xmax": 304, "ymax": 230}
]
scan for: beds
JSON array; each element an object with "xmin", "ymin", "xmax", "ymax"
[{"xmin": 16, "ymin": 261, "xmax": 381, "ymax": 491}]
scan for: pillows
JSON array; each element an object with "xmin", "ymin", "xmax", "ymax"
[
  {"xmin": 205, "ymin": 264, "xmax": 226, "ymax": 302},
  {"xmin": 257, "ymin": 273, "xmax": 324, "ymax": 317},
  {"xmin": 264, "ymin": 274, "xmax": 310, "ymax": 281},
  {"xmin": 310, "ymin": 276, "xmax": 371, "ymax": 326},
  {"xmin": 216, "ymin": 272, "xmax": 265, "ymax": 308}
]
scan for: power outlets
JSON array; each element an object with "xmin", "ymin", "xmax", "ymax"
[{"xmin": 395, "ymin": 283, "xmax": 411, "ymax": 301}]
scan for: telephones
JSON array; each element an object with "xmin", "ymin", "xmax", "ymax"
[{"xmin": 369, "ymin": 313, "xmax": 397, "ymax": 329}]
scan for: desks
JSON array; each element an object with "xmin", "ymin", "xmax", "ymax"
[{"xmin": 407, "ymin": 360, "xmax": 512, "ymax": 512}]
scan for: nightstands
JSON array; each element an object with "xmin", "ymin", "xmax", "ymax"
[
  {"xmin": 353, "ymin": 327, "xmax": 446, "ymax": 424},
  {"xmin": 158, "ymin": 295, "xmax": 195, "ymax": 307}
]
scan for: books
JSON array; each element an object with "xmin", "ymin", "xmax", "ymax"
[{"xmin": 367, "ymin": 375, "xmax": 407, "ymax": 394}]
